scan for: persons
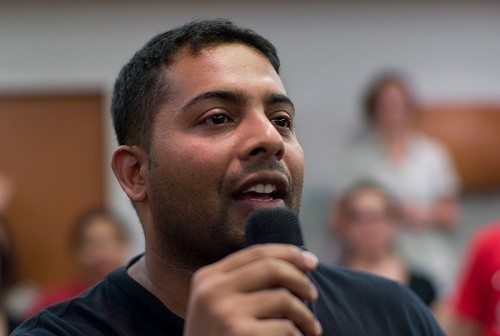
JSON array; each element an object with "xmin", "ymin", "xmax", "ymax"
[
  {"xmin": 446, "ymin": 220, "xmax": 499, "ymax": 335},
  {"xmin": 326, "ymin": 64, "xmax": 460, "ymax": 294},
  {"xmin": 9, "ymin": 16, "xmax": 445, "ymax": 335},
  {"xmin": 23, "ymin": 205, "xmax": 128, "ymax": 323},
  {"xmin": 332, "ymin": 177, "xmax": 440, "ymax": 314},
  {"xmin": 0, "ymin": 179, "xmax": 16, "ymax": 334}
]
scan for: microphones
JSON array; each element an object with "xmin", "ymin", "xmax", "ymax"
[{"xmin": 244, "ymin": 207, "xmax": 306, "ymax": 304}]
